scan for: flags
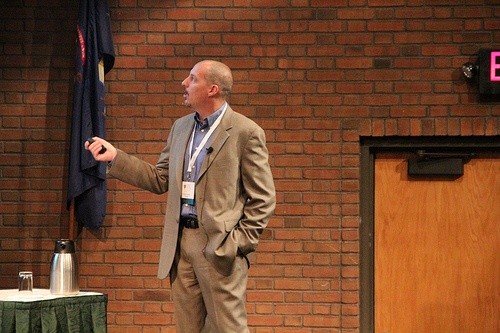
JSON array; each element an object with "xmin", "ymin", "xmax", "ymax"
[{"xmin": 67, "ymin": 0, "xmax": 115, "ymax": 233}]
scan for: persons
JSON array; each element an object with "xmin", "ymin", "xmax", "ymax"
[{"xmin": 85, "ymin": 60, "xmax": 277, "ymax": 333}]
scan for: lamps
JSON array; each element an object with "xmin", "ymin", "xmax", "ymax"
[{"xmin": 462, "ymin": 62, "xmax": 479, "ymax": 79}]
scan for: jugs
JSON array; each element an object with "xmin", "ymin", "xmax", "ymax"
[{"xmin": 49, "ymin": 240, "xmax": 79, "ymax": 294}]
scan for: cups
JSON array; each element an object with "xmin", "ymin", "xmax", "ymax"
[{"xmin": 18, "ymin": 272, "xmax": 33, "ymax": 291}]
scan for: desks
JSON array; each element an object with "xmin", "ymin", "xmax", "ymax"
[{"xmin": 0, "ymin": 289, "xmax": 109, "ymax": 333}]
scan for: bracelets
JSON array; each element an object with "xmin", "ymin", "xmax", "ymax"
[{"xmin": 106, "ymin": 161, "xmax": 113, "ymax": 168}]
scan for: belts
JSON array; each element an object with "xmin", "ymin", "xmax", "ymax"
[{"xmin": 179, "ymin": 217, "xmax": 199, "ymax": 229}]
